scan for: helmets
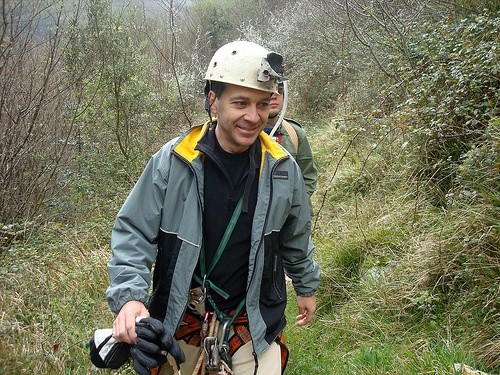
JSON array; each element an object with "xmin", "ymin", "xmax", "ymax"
[{"xmin": 202, "ymin": 40, "xmax": 279, "ymax": 96}]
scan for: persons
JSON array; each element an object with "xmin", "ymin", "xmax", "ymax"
[
  {"xmin": 104, "ymin": 39, "xmax": 321, "ymax": 375},
  {"xmin": 263, "ymin": 74, "xmax": 318, "ymax": 218}
]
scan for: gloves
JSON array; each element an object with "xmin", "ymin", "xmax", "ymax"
[{"xmin": 89, "ymin": 317, "xmax": 187, "ymax": 375}]
